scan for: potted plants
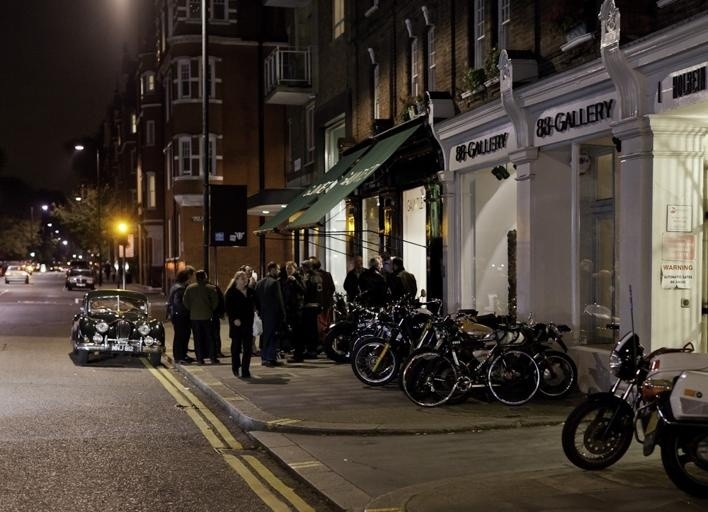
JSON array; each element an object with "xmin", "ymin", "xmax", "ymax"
[{"xmin": 462, "ymin": 66, "xmax": 488, "ymax": 92}]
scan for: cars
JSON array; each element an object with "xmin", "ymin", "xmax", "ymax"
[
  {"xmin": 67, "ymin": 289, "xmax": 167, "ymax": 368},
  {"xmin": 65, "ymin": 260, "xmax": 95, "ymax": 291},
  {"xmin": 4, "ymin": 262, "xmax": 40, "ymax": 284},
  {"xmin": 50, "ymin": 264, "xmax": 61, "ymax": 271}
]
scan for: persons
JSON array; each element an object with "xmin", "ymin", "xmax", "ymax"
[
  {"xmin": 225, "ymin": 272, "xmax": 256, "ymax": 378},
  {"xmin": 165, "ymin": 255, "xmax": 416, "ymax": 366}
]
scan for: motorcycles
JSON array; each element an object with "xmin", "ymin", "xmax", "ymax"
[
  {"xmin": 321, "ymin": 285, "xmax": 577, "ymax": 407},
  {"xmin": 558, "ymin": 323, "xmax": 695, "ymax": 472},
  {"xmin": 629, "ymin": 285, "xmax": 708, "ymax": 500}
]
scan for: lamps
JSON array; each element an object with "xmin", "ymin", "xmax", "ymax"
[{"xmin": 491, "ymin": 163, "xmax": 510, "ymax": 181}]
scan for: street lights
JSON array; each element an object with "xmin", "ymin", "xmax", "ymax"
[
  {"xmin": 86, "ymin": 248, "xmax": 99, "ymax": 281},
  {"xmin": 117, "ymin": 220, "xmax": 128, "ymax": 290},
  {"xmin": 30, "ymin": 205, "xmax": 49, "ymax": 260},
  {"xmin": 73, "ymin": 141, "xmax": 103, "ymax": 261},
  {"xmin": 75, "ymin": 196, "xmax": 103, "ymax": 284}
]
